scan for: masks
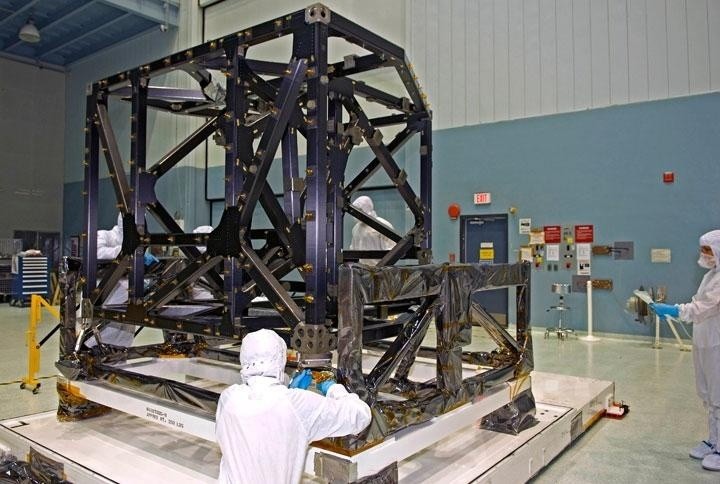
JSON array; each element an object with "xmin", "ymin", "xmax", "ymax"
[{"xmin": 697, "ymin": 254, "xmax": 716, "ymax": 269}]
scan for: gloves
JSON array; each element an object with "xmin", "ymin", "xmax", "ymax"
[
  {"xmin": 316, "ymin": 376, "xmax": 336, "ymax": 394},
  {"xmin": 648, "ymin": 302, "xmax": 679, "ymax": 321},
  {"xmin": 287, "ymin": 369, "xmax": 313, "ymax": 390}
]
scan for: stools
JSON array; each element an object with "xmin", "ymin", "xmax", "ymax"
[{"xmin": 544, "ymin": 283, "xmax": 577, "ymax": 341}]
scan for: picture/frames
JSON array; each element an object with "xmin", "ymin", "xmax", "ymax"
[{"xmin": 71, "ymin": 236, "xmax": 80, "ymax": 257}]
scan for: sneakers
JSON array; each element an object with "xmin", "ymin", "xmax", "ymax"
[{"xmin": 688, "ymin": 440, "xmax": 719, "ymax": 472}]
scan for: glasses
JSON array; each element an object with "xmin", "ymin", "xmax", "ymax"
[{"xmin": 696, "ymin": 247, "xmax": 712, "ymax": 253}]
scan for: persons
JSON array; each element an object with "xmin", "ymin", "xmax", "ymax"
[
  {"xmin": 213, "ymin": 326, "xmax": 373, "ymax": 484},
  {"xmin": 188, "ymin": 224, "xmax": 225, "ymax": 301},
  {"xmin": 347, "ymin": 194, "xmax": 398, "ymax": 265},
  {"xmin": 649, "ymin": 226, "xmax": 720, "ymax": 471},
  {"xmin": 80, "ymin": 211, "xmax": 161, "ymax": 350}
]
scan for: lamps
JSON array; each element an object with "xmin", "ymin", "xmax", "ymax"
[{"xmin": 19, "ymin": 9, "xmax": 41, "ymax": 42}]
